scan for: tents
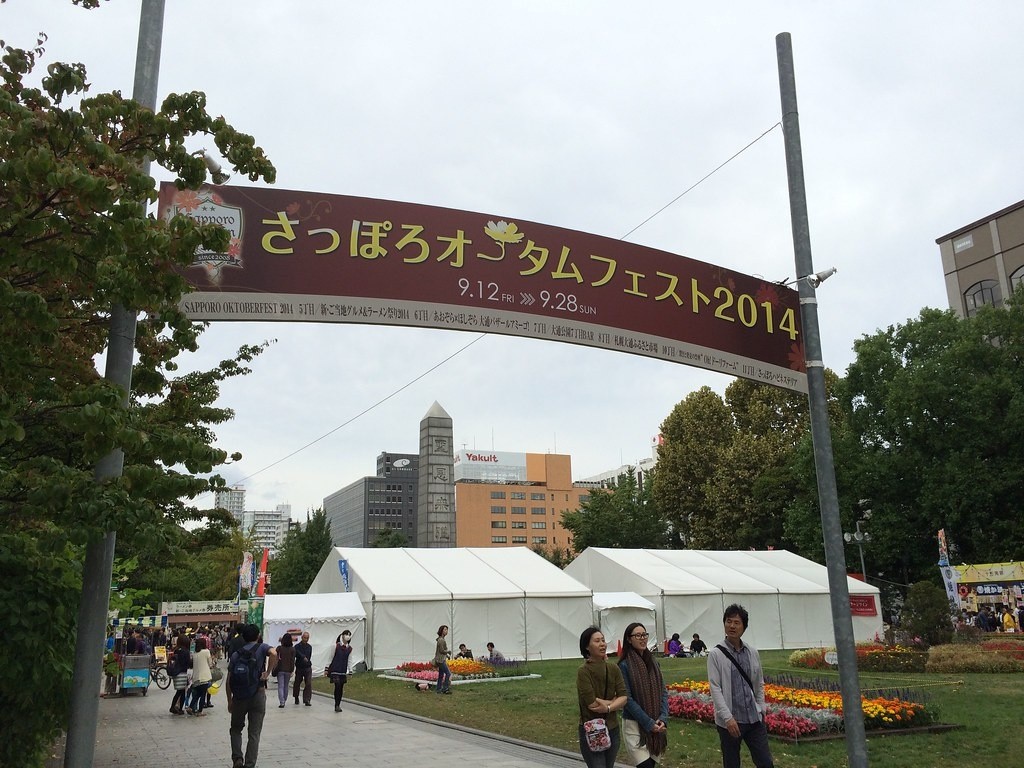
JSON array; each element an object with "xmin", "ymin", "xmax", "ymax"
[
  {"xmin": 564, "ymin": 547, "xmax": 884, "ymax": 651},
  {"xmin": 263, "ymin": 591, "xmax": 367, "ymax": 681},
  {"xmin": 592, "ymin": 591, "xmax": 658, "ymax": 654},
  {"xmin": 305, "ymin": 546, "xmax": 594, "ymax": 670}
]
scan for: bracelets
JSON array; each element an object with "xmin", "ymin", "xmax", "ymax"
[{"xmin": 608, "ymin": 705, "xmax": 610, "ymax": 714}]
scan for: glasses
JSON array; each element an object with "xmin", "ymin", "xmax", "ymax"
[{"xmin": 632, "ymin": 633, "xmax": 649, "ymax": 640}]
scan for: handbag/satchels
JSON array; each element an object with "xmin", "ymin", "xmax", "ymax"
[
  {"xmin": 584, "ymin": 718, "xmax": 611, "ymax": 753},
  {"xmin": 272, "ymin": 661, "xmax": 281, "ymax": 677},
  {"xmin": 211, "ymin": 667, "xmax": 222, "ymax": 681},
  {"xmin": 207, "ymin": 682, "xmax": 220, "ymax": 695}
]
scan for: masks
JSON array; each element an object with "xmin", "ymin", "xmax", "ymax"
[{"xmin": 344, "ymin": 636, "xmax": 350, "ymax": 641}]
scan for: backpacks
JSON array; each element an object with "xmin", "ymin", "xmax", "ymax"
[
  {"xmin": 233, "ymin": 643, "xmax": 261, "ymax": 688},
  {"xmin": 167, "ymin": 655, "xmax": 181, "ymax": 676}
]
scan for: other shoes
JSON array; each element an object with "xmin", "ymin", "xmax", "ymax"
[
  {"xmin": 178, "ymin": 710, "xmax": 185, "ymax": 714},
  {"xmin": 279, "ymin": 703, "xmax": 285, "ymax": 708},
  {"xmin": 170, "ymin": 707, "xmax": 178, "ymax": 714},
  {"xmin": 443, "ymin": 689, "xmax": 451, "ymax": 694},
  {"xmin": 187, "ymin": 708, "xmax": 194, "ymax": 715},
  {"xmin": 208, "ymin": 702, "xmax": 214, "ymax": 707},
  {"xmin": 304, "ymin": 701, "xmax": 311, "ymax": 706},
  {"xmin": 204, "ymin": 704, "xmax": 207, "ymax": 708},
  {"xmin": 335, "ymin": 706, "xmax": 342, "ymax": 713},
  {"xmin": 295, "ymin": 698, "xmax": 299, "ymax": 704},
  {"xmin": 196, "ymin": 712, "xmax": 207, "ymax": 717}
]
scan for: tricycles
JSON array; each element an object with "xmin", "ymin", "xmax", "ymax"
[{"xmin": 117, "ymin": 654, "xmax": 151, "ymax": 697}]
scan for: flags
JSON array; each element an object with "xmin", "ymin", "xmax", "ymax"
[
  {"xmin": 338, "ymin": 559, "xmax": 349, "ymax": 591},
  {"xmin": 231, "ymin": 548, "xmax": 268, "ymax": 606},
  {"xmin": 937, "ymin": 529, "xmax": 948, "ymax": 566}
]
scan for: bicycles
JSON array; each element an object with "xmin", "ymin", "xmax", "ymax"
[{"xmin": 150, "ymin": 659, "xmax": 171, "ymax": 690}]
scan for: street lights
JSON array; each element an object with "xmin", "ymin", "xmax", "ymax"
[{"xmin": 844, "ymin": 521, "xmax": 870, "ymax": 582}]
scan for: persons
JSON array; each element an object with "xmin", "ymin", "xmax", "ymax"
[
  {"xmin": 226, "ymin": 624, "xmax": 277, "ymax": 768},
  {"xmin": 952, "ymin": 604, "xmax": 1024, "ymax": 633},
  {"xmin": 707, "ymin": 602, "xmax": 774, "ymax": 768},
  {"xmin": 276, "ymin": 630, "xmax": 353, "ymax": 711},
  {"xmin": 616, "ymin": 623, "xmax": 670, "ymax": 768},
  {"xmin": 454, "ymin": 644, "xmax": 472, "ymax": 660},
  {"xmin": 576, "ymin": 627, "xmax": 628, "ymax": 768},
  {"xmin": 435, "ymin": 625, "xmax": 452, "ymax": 694},
  {"xmin": 104, "ymin": 622, "xmax": 264, "ymax": 716},
  {"xmin": 667, "ymin": 633, "xmax": 708, "ymax": 658},
  {"xmin": 487, "ymin": 642, "xmax": 505, "ymax": 664}
]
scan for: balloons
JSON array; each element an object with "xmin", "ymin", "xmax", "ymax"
[{"xmin": 208, "ymin": 683, "xmax": 219, "ymax": 695}]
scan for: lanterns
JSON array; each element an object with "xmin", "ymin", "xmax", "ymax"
[{"xmin": 959, "ymin": 586, "xmax": 968, "ymax": 597}]
